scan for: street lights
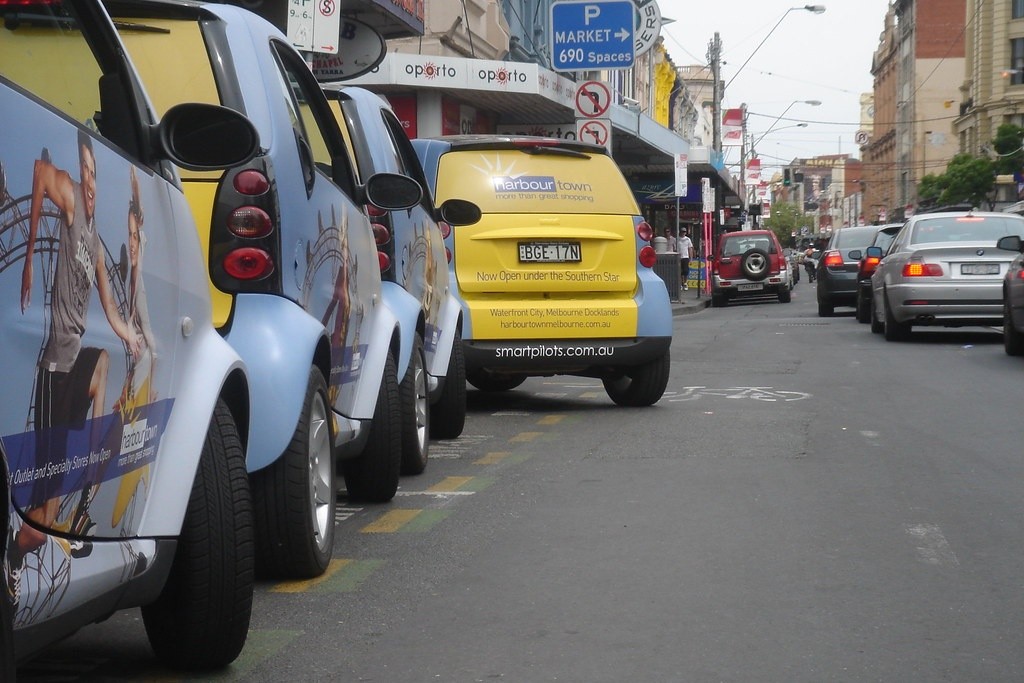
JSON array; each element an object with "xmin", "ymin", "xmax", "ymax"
[
  {"xmin": 712, "ymin": 4, "xmax": 828, "ymax": 155},
  {"xmin": 726, "ymin": 100, "xmax": 823, "ymax": 170},
  {"xmin": 752, "ymin": 122, "xmax": 809, "ymax": 160}
]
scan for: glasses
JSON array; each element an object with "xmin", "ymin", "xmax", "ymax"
[
  {"xmin": 683, "ymin": 231, "xmax": 686, "ymax": 232},
  {"xmin": 665, "ymin": 231, "xmax": 670, "ymax": 233}
]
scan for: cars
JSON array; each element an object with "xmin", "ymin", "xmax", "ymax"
[
  {"xmin": 408, "ymin": 133, "xmax": 674, "ymax": 408},
  {"xmin": 0, "ymin": 0, "xmax": 262, "ymax": 666},
  {"xmin": 0, "ymin": 0, "xmax": 424, "ymax": 583},
  {"xmin": 996, "ymin": 235, "xmax": 1024, "ymax": 356},
  {"xmin": 783, "ymin": 248, "xmax": 805, "ymax": 290},
  {"xmin": 867, "ymin": 207, "xmax": 1024, "ymax": 341},
  {"xmin": 285, "ymin": 81, "xmax": 483, "ymax": 476}
]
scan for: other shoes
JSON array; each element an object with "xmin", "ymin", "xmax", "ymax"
[
  {"xmin": 681, "ymin": 286, "xmax": 683, "ymax": 290},
  {"xmin": 683, "ymin": 283, "xmax": 688, "ymax": 290}
]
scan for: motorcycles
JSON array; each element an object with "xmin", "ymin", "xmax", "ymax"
[{"xmin": 805, "ymin": 252, "xmax": 816, "ymax": 284}]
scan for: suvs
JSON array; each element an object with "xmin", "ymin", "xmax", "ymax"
[
  {"xmin": 811, "ymin": 225, "xmax": 885, "ymax": 317},
  {"xmin": 847, "ymin": 222, "xmax": 907, "ymax": 324},
  {"xmin": 706, "ymin": 229, "xmax": 791, "ymax": 307}
]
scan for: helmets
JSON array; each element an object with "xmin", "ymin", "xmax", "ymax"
[{"xmin": 809, "ymin": 243, "xmax": 814, "ymax": 245}]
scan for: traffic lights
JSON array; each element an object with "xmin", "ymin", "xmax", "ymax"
[{"xmin": 784, "ymin": 168, "xmax": 790, "ymax": 186}]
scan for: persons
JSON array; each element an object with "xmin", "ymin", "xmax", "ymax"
[
  {"xmin": 803, "ymin": 242, "xmax": 817, "ymax": 256},
  {"xmin": 664, "ymin": 227, "xmax": 676, "ymax": 252},
  {"xmin": 677, "ymin": 227, "xmax": 695, "ymax": 291}
]
